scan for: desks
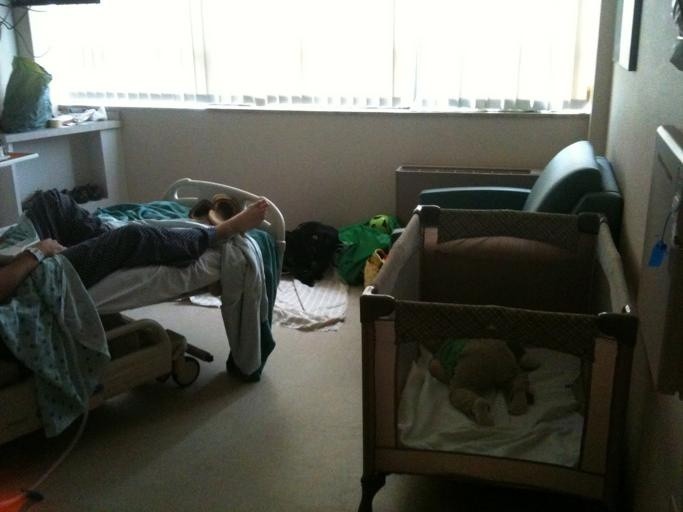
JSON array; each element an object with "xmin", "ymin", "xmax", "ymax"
[
  {"xmin": 0, "ymin": 118, "xmax": 130, "ymax": 223},
  {"xmin": 0, "ymin": 151, "xmax": 38, "ymax": 169}
]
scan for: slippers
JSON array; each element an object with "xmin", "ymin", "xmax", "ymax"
[{"xmin": 188, "ymin": 193, "xmax": 239, "ymax": 226}]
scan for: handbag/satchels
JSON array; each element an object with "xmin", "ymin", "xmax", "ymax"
[
  {"xmin": 363, "ymin": 248, "xmax": 386, "ymax": 289},
  {"xmin": 287, "ymin": 222, "xmax": 338, "ymax": 281}
]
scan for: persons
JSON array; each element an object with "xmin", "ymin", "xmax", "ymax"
[
  {"xmin": 0, "ymin": 186, "xmax": 269, "ymax": 304},
  {"xmin": 419, "ymin": 324, "xmax": 538, "ymax": 426}
]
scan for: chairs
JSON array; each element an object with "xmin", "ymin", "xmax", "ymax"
[{"xmin": 389, "ymin": 140, "xmax": 625, "ymax": 249}]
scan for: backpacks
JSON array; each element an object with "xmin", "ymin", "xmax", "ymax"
[{"xmin": 26, "ymin": 188, "xmax": 101, "ymax": 247}]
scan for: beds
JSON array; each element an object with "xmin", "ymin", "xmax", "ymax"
[
  {"xmin": 358, "ymin": 203, "xmax": 640, "ymax": 512},
  {"xmin": 0, "ymin": 177, "xmax": 287, "ymax": 447}
]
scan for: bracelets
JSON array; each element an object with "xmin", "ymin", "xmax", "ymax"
[{"xmin": 26, "ymin": 245, "xmax": 46, "ymax": 264}]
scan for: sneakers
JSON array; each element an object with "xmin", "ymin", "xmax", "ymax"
[{"xmin": 62, "ymin": 186, "xmax": 100, "ymax": 203}]
